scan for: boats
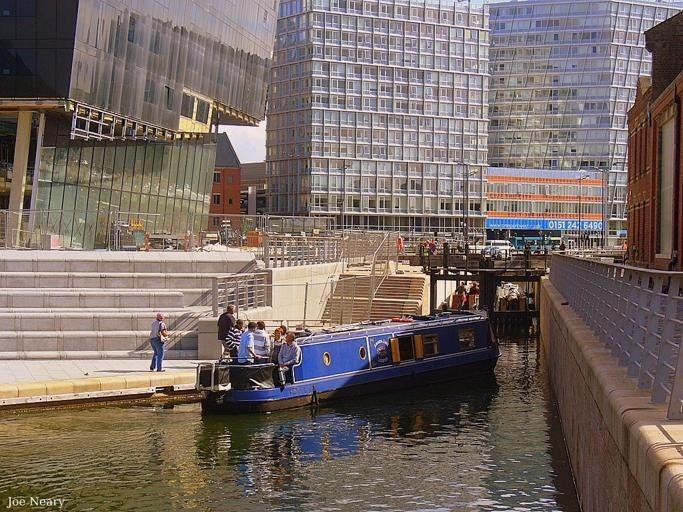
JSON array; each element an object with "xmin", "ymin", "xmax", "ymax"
[
  {"xmin": 196, "ymin": 376, "xmax": 499, "ymax": 494},
  {"xmin": 184, "ymin": 308, "xmax": 507, "ymax": 415},
  {"xmin": 492, "ymin": 282, "xmax": 529, "ymax": 319}
]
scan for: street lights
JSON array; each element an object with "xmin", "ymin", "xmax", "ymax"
[
  {"xmin": 461, "ymin": 168, "xmax": 479, "ymax": 240},
  {"xmin": 576, "ymin": 174, "xmax": 590, "ymax": 249},
  {"xmin": 287, "ymin": 153, "xmax": 300, "ymax": 217}
]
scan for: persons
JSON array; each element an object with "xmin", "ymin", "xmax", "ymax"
[
  {"xmin": 469, "ymin": 283, "xmax": 479, "ymax": 295},
  {"xmin": 525, "ymin": 243, "xmax": 531, "ymax": 249},
  {"xmin": 218, "ymin": 304, "xmax": 235, "ymax": 354},
  {"xmin": 225, "ymin": 319, "xmax": 301, "ymax": 382},
  {"xmin": 507, "ymin": 288, "xmax": 520, "ymax": 312},
  {"xmin": 559, "ymin": 242, "xmax": 565, "ymax": 254},
  {"xmin": 149, "ymin": 313, "xmax": 170, "ymax": 372},
  {"xmin": 455, "ymin": 282, "xmax": 467, "ymax": 307}
]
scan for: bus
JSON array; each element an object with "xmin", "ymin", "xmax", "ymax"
[{"xmin": 511, "ymin": 236, "xmax": 560, "ymax": 253}]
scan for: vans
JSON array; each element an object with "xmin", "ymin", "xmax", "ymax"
[{"xmin": 482, "ymin": 239, "xmax": 516, "ymax": 253}]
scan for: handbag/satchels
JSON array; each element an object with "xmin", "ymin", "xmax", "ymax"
[{"xmin": 159, "ymin": 330, "xmax": 170, "ymax": 343}]
